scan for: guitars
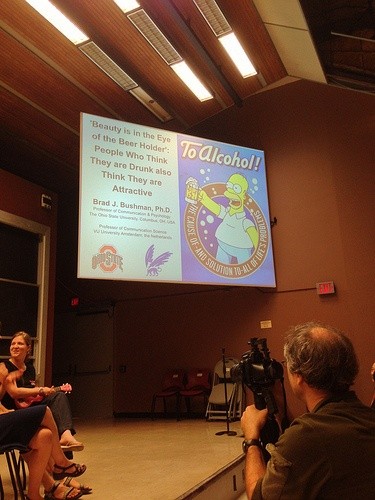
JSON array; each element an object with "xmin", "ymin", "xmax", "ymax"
[{"xmin": 15, "ymin": 383, "xmax": 72, "ymax": 408}]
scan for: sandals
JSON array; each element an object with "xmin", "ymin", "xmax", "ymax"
[
  {"xmin": 52, "ymin": 462, "xmax": 87, "ymax": 480},
  {"xmin": 63, "ymin": 477, "xmax": 94, "ymax": 493},
  {"xmin": 44, "ymin": 481, "xmax": 83, "ymax": 499}
]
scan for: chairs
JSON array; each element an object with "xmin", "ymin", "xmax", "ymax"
[{"xmin": 151, "ymin": 357, "xmax": 243, "ymax": 422}]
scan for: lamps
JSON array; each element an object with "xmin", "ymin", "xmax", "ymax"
[{"xmin": 25, "ymin": 0, "xmax": 259, "ymax": 124}]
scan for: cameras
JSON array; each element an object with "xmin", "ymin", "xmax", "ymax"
[{"xmin": 229, "ymin": 338, "xmax": 284, "ymax": 385}]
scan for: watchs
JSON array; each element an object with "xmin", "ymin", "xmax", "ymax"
[{"xmin": 242, "ymin": 439, "xmax": 262, "ymax": 454}]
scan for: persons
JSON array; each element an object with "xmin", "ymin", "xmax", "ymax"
[
  {"xmin": 241, "ymin": 322, "xmax": 375, "ymax": 500},
  {"xmin": 0, "ymin": 333, "xmax": 92, "ymax": 500}
]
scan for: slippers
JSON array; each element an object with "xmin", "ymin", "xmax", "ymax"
[{"xmin": 61, "ymin": 438, "xmax": 84, "ymax": 451}]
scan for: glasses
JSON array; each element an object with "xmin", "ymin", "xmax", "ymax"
[{"xmin": 282, "ymin": 360, "xmax": 289, "ymax": 368}]
